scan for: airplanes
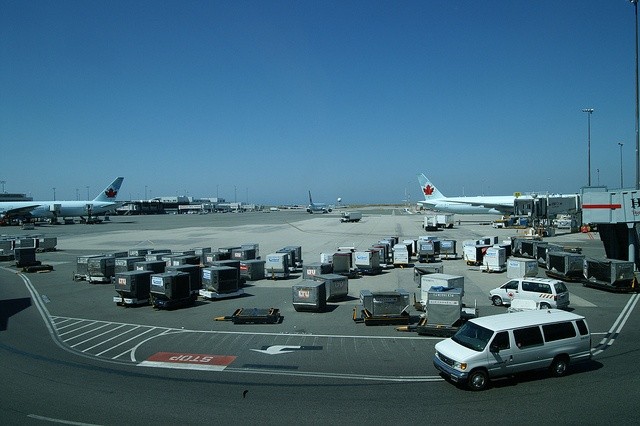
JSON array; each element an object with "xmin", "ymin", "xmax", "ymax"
[
  {"xmin": 0, "ymin": 177, "xmax": 124, "ymax": 223},
  {"xmin": 417, "ymin": 174, "xmax": 581, "ymax": 221},
  {"xmin": 304, "ymin": 190, "xmax": 347, "ymax": 214}
]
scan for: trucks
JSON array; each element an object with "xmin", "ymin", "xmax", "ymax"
[
  {"xmin": 437, "ymin": 213, "xmax": 454, "ymax": 228},
  {"xmin": 340, "ymin": 212, "xmax": 362, "ymax": 223},
  {"xmin": 422, "ymin": 216, "xmax": 437, "ymax": 231}
]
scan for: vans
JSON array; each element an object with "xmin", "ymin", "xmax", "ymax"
[
  {"xmin": 489, "ymin": 277, "xmax": 570, "ymax": 310},
  {"xmin": 433, "ymin": 308, "xmax": 592, "ymax": 391}
]
